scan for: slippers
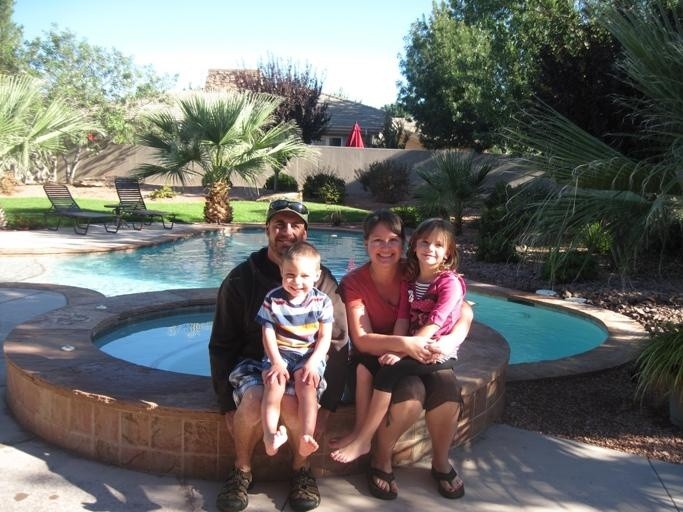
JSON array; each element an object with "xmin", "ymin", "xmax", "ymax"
[
  {"xmin": 367, "ymin": 456, "xmax": 398, "ymax": 499},
  {"xmin": 431, "ymin": 464, "xmax": 464, "ymax": 498}
]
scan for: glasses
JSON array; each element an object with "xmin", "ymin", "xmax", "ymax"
[{"xmin": 270, "ymin": 200, "xmax": 308, "ymax": 214}]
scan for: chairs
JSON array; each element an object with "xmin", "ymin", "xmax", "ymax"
[{"xmin": 42, "ymin": 178, "xmax": 176, "ymax": 235}]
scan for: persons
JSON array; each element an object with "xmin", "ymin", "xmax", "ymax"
[
  {"xmin": 337, "ymin": 206, "xmax": 474, "ymax": 503},
  {"xmin": 326, "ymin": 217, "xmax": 465, "ymax": 465},
  {"xmin": 205, "ymin": 193, "xmax": 347, "ymax": 510},
  {"xmin": 251, "ymin": 241, "xmax": 334, "ymax": 458}
]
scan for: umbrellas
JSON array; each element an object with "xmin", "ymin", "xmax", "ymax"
[{"xmin": 346, "ymin": 123, "xmax": 363, "ymax": 147}]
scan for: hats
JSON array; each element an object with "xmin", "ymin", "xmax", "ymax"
[{"xmin": 266, "ymin": 198, "xmax": 309, "ymax": 228}]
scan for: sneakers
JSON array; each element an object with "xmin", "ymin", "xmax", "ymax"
[
  {"xmin": 289, "ymin": 467, "xmax": 320, "ymax": 511},
  {"xmin": 216, "ymin": 464, "xmax": 255, "ymax": 512}
]
